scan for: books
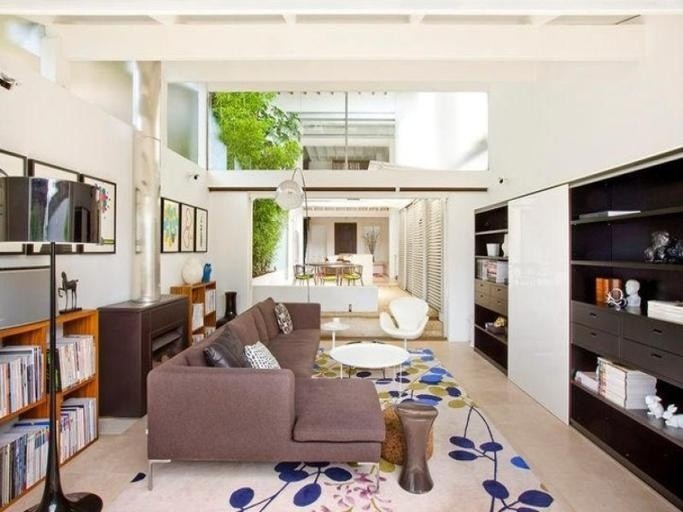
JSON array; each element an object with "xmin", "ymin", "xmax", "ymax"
[
  {"xmin": 0, "ymin": 334, "xmax": 98, "ymax": 508},
  {"xmin": 578, "ymin": 210, "xmax": 641, "ymax": 221},
  {"xmin": 646, "ymin": 298, "xmax": 682, "ymax": 323},
  {"xmin": 191, "ymin": 290, "xmax": 215, "ymax": 343},
  {"xmin": 476, "ymin": 259, "xmax": 507, "ymax": 284},
  {"xmin": 573, "ymin": 355, "xmax": 657, "ymax": 411}
]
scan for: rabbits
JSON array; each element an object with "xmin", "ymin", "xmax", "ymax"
[
  {"xmin": 644, "ymin": 395, "xmax": 664, "ymax": 419},
  {"xmin": 662, "ymin": 403, "xmax": 683, "ymax": 428}
]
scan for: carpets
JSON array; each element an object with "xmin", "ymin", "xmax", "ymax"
[{"xmin": 102, "ymin": 347, "xmax": 562, "ymax": 511}]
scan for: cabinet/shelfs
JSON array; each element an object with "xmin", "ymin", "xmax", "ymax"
[
  {"xmin": 98, "ymin": 292, "xmax": 189, "ymax": 417},
  {"xmin": 171, "ymin": 281, "xmax": 217, "ymax": 343},
  {"xmin": 568, "ymin": 147, "xmax": 683, "ymax": 511},
  {"xmin": 0, "ymin": 308, "xmax": 99, "ymax": 511},
  {"xmin": 472, "ymin": 201, "xmax": 508, "ymax": 377}
]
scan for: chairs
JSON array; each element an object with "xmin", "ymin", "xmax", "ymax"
[
  {"xmin": 379, "ymin": 299, "xmax": 428, "ymax": 352},
  {"xmin": 293, "ymin": 259, "xmax": 364, "ymax": 287}
]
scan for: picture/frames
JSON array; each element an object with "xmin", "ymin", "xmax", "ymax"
[
  {"xmin": 28, "ymin": 158, "xmax": 79, "ymax": 254},
  {"xmin": 0, "ymin": 148, "xmax": 28, "ymax": 254},
  {"xmin": 160, "ymin": 196, "xmax": 208, "ymax": 252},
  {"xmin": 79, "ymin": 174, "xmax": 117, "ymax": 254}
]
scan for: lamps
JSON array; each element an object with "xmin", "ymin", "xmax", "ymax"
[{"xmin": 275, "ymin": 167, "xmax": 310, "ymax": 303}]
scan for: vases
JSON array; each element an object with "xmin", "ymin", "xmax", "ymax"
[{"xmin": 216, "ymin": 291, "xmax": 238, "ymax": 331}]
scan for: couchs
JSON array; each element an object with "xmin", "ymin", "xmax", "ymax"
[{"xmin": 147, "ymin": 298, "xmax": 385, "ymax": 490}]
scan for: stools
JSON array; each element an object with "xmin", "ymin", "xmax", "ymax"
[
  {"xmin": 395, "ymin": 402, "xmax": 437, "ymax": 494},
  {"xmin": 380, "ymin": 407, "xmax": 432, "ymax": 465}
]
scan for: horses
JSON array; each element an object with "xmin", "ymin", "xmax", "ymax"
[{"xmin": 57, "ymin": 271, "xmax": 78, "ymax": 311}]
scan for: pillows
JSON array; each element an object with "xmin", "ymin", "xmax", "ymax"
[
  {"xmin": 202, "ymin": 325, "xmax": 249, "ymax": 367},
  {"xmin": 243, "ymin": 340, "xmax": 281, "ymax": 368},
  {"xmin": 273, "ymin": 303, "xmax": 293, "ymax": 334}
]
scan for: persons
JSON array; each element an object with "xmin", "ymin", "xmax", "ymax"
[{"xmin": 624, "ymin": 279, "xmax": 640, "ymax": 308}]
